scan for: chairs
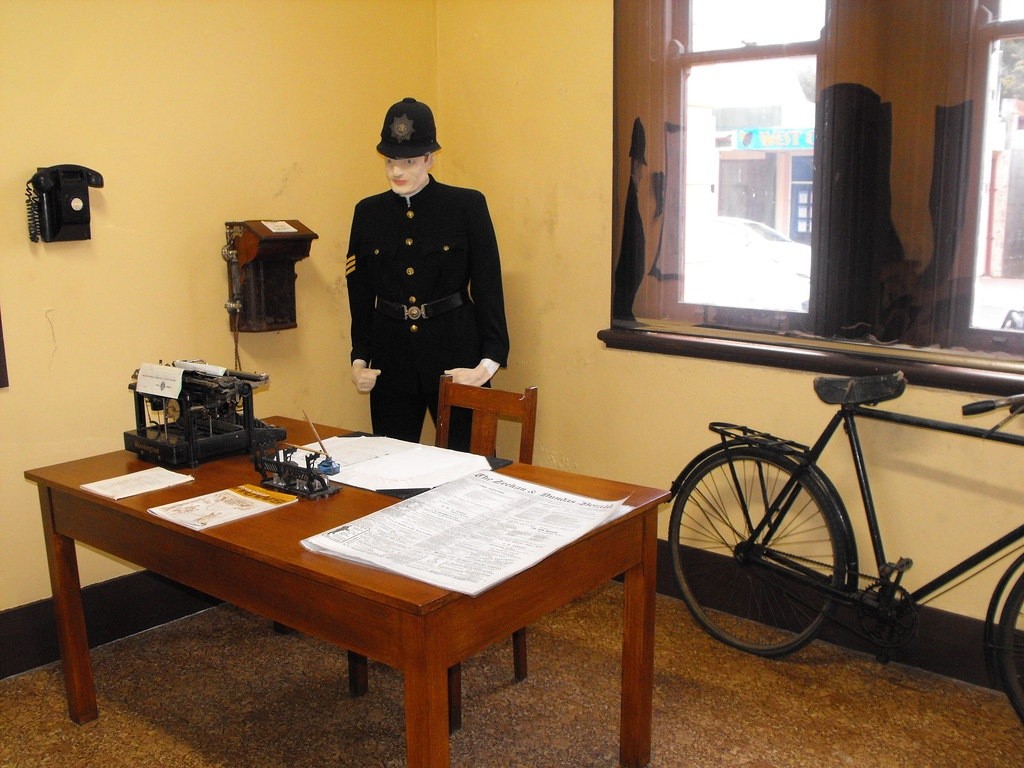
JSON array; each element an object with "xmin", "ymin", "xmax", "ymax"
[{"xmin": 348, "ymin": 374, "xmax": 539, "ymax": 733}]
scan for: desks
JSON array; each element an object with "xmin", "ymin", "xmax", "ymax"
[{"xmin": 24, "ymin": 415, "xmax": 673, "ymax": 768}]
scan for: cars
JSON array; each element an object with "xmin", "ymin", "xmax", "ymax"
[{"xmin": 718, "ymin": 216, "xmax": 812, "ymax": 282}]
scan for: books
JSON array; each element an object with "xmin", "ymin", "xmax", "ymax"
[
  {"xmin": 79, "ymin": 466, "xmax": 195, "ymax": 501},
  {"xmin": 146, "ymin": 484, "xmax": 299, "ymax": 532}
]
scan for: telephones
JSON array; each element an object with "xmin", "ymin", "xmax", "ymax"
[
  {"xmin": 30, "ymin": 163, "xmax": 103, "ymax": 241},
  {"xmin": 222, "ymin": 216, "xmax": 319, "ymax": 333}
]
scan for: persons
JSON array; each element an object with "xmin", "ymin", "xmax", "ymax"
[{"xmin": 344, "ymin": 97, "xmax": 510, "ymax": 457}]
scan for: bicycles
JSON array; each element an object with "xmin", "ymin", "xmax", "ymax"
[{"xmin": 662, "ymin": 370, "xmax": 1024, "ymax": 730}]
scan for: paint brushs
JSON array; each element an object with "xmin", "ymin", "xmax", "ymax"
[
  {"xmin": 302, "ymin": 410, "xmax": 331, "ymax": 459},
  {"xmin": 283, "ymin": 442, "xmax": 329, "ymax": 457}
]
